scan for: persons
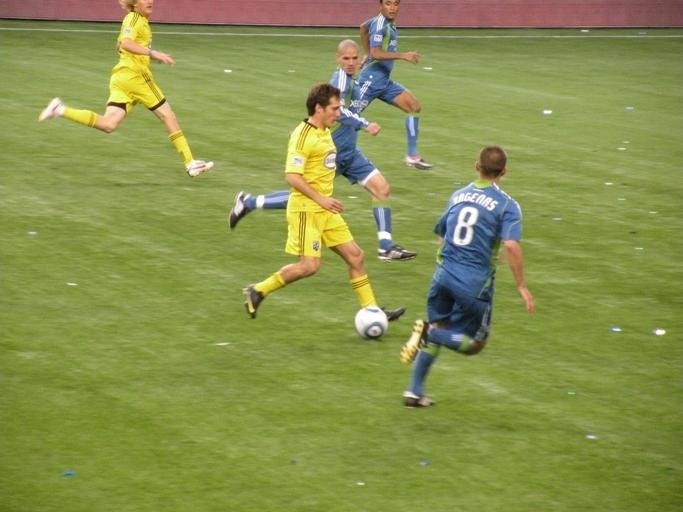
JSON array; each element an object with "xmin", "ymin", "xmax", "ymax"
[
  {"xmin": 347, "ymin": 0, "xmax": 436, "ymax": 173},
  {"xmin": 398, "ymin": 144, "xmax": 536, "ymax": 409},
  {"xmin": 36, "ymin": 0, "xmax": 215, "ymax": 182},
  {"xmin": 227, "ymin": 38, "xmax": 418, "ymax": 265},
  {"xmin": 242, "ymin": 81, "xmax": 407, "ymax": 325}
]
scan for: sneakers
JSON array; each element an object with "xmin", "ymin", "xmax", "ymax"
[
  {"xmin": 405, "ymin": 156, "xmax": 434, "ymax": 170},
  {"xmin": 38, "ymin": 96, "xmax": 63, "ymax": 124},
  {"xmin": 382, "ymin": 305, "xmax": 407, "ymax": 322},
  {"xmin": 400, "ymin": 390, "xmax": 435, "ymax": 408},
  {"xmin": 241, "ymin": 282, "xmax": 265, "ymax": 319},
  {"xmin": 186, "ymin": 159, "xmax": 214, "ymax": 178},
  {"xmin": 376, "ymin": 243, "xmax": 417, "ymax": 263},
  {"xmin": 399, "ymin": 318, "xmax": 431, "ymax": 366},
  {"xmin": 228, "ymin": 189, "xmax": 252, "ymax": 230}
]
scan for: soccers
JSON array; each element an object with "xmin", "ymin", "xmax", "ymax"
[{"xmin": 355, "ymin": 307, "xmax": 388, "ymax": 339}]
